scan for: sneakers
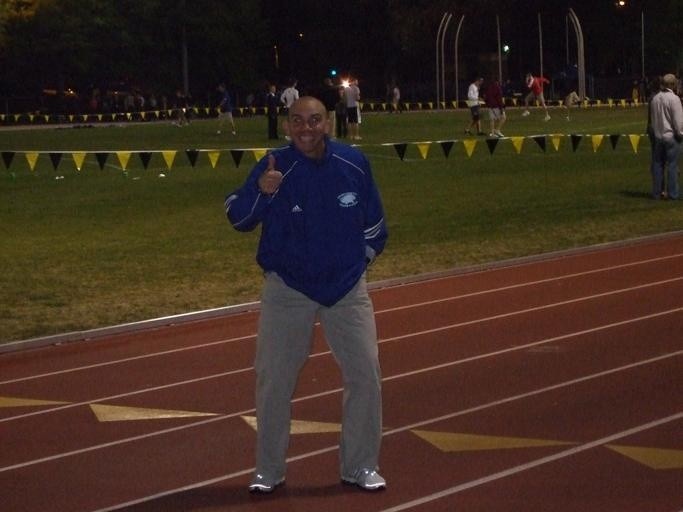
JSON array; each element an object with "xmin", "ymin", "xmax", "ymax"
[
  {"xmin": 342, "ymin": 467, "xmax": 386, "ymax": 490},
  {"xmin": 248, "ymin": 474, "xmax": 285, "ymax": 491}
]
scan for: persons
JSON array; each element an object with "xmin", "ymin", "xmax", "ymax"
[
  {"xmin": 216, "ymin": 84, "xmax": 236, "ymax": 136},
  {"xmin": 646, "ymin": 73, "xmax": 683, "ymax": 200},
  {"xmin": 486, "ymin": 75, "xmax": 507, "ymax": 138},
  {"xmin": 224, "ymin": 96, "xmax": 390, "ymax": 493},
  {"xmin": 464, "ymin": 77, "xmax": 487, "ymax": 136},
  {"xmin": 390, "ymin": 80, "xmax": 401, "ymax": 103},
  {"xmin": 648, "ymin": 76, "xmax": 664, "ymax": 181},
  {"xmin": 265, "ymin": 77, "xmax": 363, "ymax": 140},
  {"xmin": 565, "ymin": 91, "xmax": 580, "ymax": 122},
  {"xmin": 522, "ymin": 73, "xmax": 552, "ymax": 122}
]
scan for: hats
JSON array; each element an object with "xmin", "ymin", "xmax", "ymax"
[{"xmin": 660, "ymin": 74, "xmax": 679, "ymax": 90}]
